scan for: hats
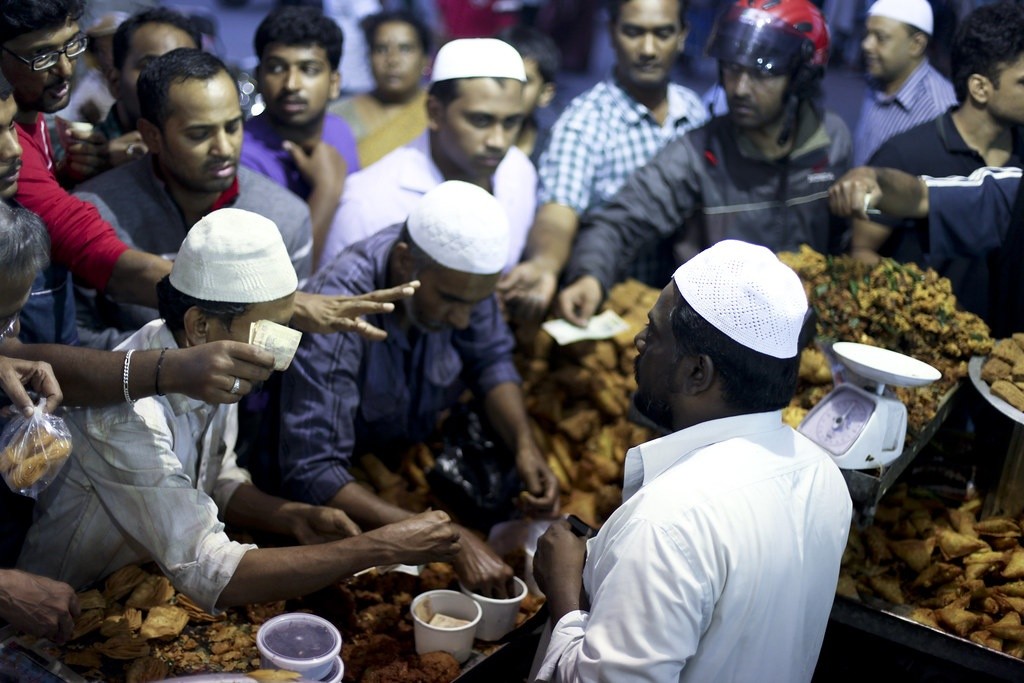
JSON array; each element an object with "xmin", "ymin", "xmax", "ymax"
[
  {"xmin": 169, "ymin": 208, "xmax": 299, "ymax": 302},
  {"xmin": 671, "ymin": 239, "xmax": 809, "ymax": 360},
  {"xmin": 430, "ymin": 38, "xmax": 528, "ymax": 85},
  {"xmin": 406, "ymin": 180, "xmax": 509, "ymax": 276},
  {"xmin": 867, "ymin": 0, "xmax": 936, "ymax": 35}
]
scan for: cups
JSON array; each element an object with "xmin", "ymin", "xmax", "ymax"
[
  {"xmin": 460, "ymin": 575, "xmax": 529, "ymax": 642},
  {"xmin": 410, "ymin": 589, "xmax": 482, "ymax": 665}
]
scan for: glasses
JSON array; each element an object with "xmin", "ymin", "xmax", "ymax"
[{"xmin": 2, "ymin": 30, "xmax": 88, "ymax": 71}]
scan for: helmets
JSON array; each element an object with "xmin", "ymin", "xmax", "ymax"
[{"xmin": 704, "ymin": 0, "xmax": 831, "ymax": 98}]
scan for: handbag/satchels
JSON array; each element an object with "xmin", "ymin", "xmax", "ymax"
[{"xmin": 0, "ymin": 392, "xmax": 73, "ymax": 499}]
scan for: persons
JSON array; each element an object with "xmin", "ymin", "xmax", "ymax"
[
  {"xmin": 23, "ymin": 208, "xmax": 460, "ymax": 601},
  {"xmin": 558, "ymin": 0, "xmax": 851, "ymax": 329},
  {"xmin": 495, "ymin": 0, "xmax": 712, "ymax": 319},
  {"xmin": 326, "ymin": 10, "xmax": 435, "ymax": 170},
  {"xmin": 314, "ymin": 38, "xmax": 539, "ymax": 277},
  {"xmin": 531, "ymin": 239, "xmax": 853, "ymax": 683},
  {"xmin": 238, "ymin": 7, "xmax": 359, "ymax": 276},
  {"xmin": 0, "ymin": 0, "xmax": 314, "ymax": 410},
  {"xmin": 852, "ymin": 0, "xmax": 959, "ymax": 168},
  {"xmin": 504, "ymin": 23, "xmax": 564, "ymax": 172},
  {"xmin": 281, "ymin": 180, "xmax": 559, "ymax": 599},
  {"xmin": 850, "ymin": 0, "xmax": 1024, "ymax": 266},
  {"xmin": 828, "ymin": 165, "xmax": 1024, "ymax": 340},
  {"xmin": 0, "ymin": 356, "xmax": 81, "ymax": 628}
]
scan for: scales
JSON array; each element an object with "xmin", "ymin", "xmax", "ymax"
[{"xmin": 796, "ymin": 339, "xmax": 944, "ymax": 469}]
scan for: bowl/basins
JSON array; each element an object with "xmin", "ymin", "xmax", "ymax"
[
  {"xmin": 318, "ymin": 656, "xmax": 345, "ymax": 683},
  {"xmin": 256, "ymin": 612, "xmax": 342, "ymax": 681}
]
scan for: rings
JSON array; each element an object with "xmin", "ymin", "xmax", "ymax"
[{"xmin": 230, "ymin": 377, "xmax": 241, "ymax": 394}]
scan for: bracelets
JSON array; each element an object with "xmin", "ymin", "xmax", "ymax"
[
  {"xmin": 155, "ymin": 347, "xmax": 168, "ymax": 396},
  {"xmin": 123, "ymin": 348, "xmax": 136, "ymax": 408}
]
